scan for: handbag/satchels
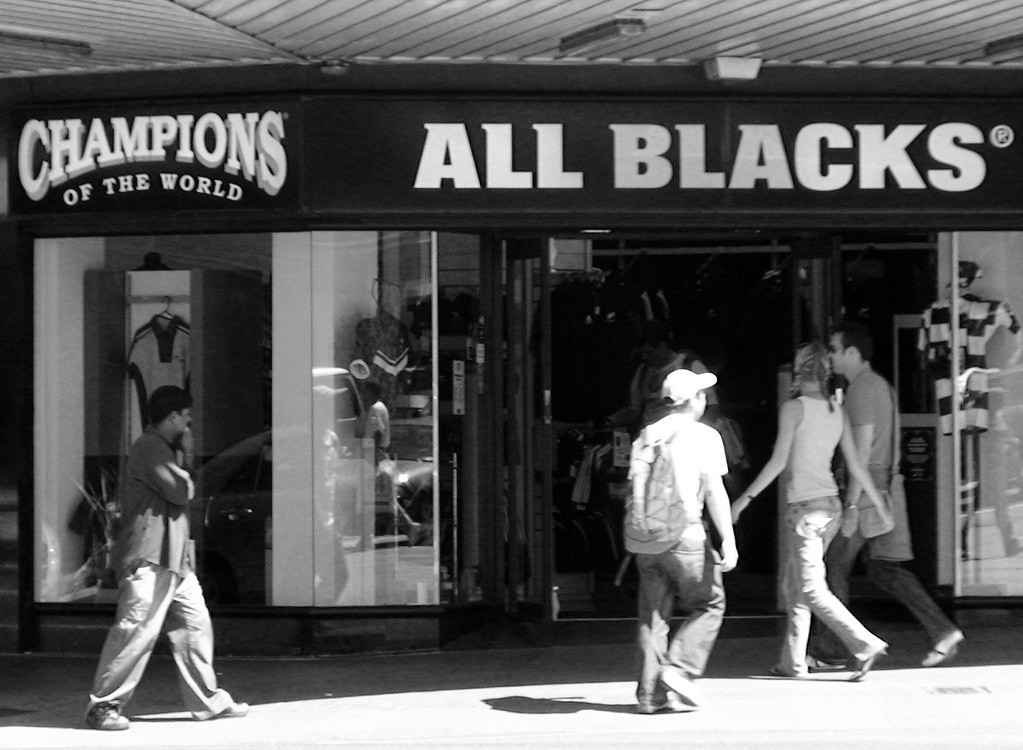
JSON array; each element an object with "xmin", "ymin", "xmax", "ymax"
[{"xmin": 856, "ymin": 489, "xmax": 895, "ymax": 538}]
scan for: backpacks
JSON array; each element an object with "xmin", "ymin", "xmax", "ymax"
[{"xmin": 623, "ymin": 424, "xmax": 687, "ymax": 553}]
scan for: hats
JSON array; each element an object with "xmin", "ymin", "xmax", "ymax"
[{"xmin": 661, "ymin": 369, "xmax": 718, "ymax": 407}]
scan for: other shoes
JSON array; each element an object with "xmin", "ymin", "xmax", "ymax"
[
  {"xmin": 661, "ymin": 664, "xmax": 699, "ymax": 706},
  {"xmin": 87, "ymin": 701, "xmax": 130, "ymax": 730},
  {"xmin": 638, "ymin": 692, "xmax": 669, "ymax": 713},
  {"xmin": 210, "ymin": 697, "xmax": 248, "ymax": 717},
  {"xmin": 766, "ymin": 662, "xmax": 788, "ymax": 676},
  {"xmin": 849, "ymin": 651, "xmax": 883, "ymax": 681},
  {"xmin": 922, "ymin": 631, "xmax": 964, "ymax": 666},
  {"xmin": 805, "ymin": 654, "xmax": 846, "ymax": 670}
]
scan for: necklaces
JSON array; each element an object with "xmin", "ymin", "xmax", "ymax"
[{"xmin": 804, "ymin": 389, "xmax": 822, "ymax": 395}]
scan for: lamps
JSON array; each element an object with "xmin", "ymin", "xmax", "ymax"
[
  {"xmin": 560, "ymin": 18, "xmax": 646, "ymax": 58},
  {"xmin": 703, "ymin": 56, "xmax": 762, "ymax": 82}
]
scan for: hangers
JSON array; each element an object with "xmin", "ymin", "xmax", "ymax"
[{"xmin": 159, "ymin": 296, "xmax": 174, "ymax": 316}]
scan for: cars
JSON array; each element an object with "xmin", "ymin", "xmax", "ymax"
[{"xmin": 336, "ymin": 418, "xmax": 435, "ymax": 530}]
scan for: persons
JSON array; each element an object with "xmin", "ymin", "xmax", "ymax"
[
  {"xmin": 605, "ymin": 320, "xmax": 720, "ymax": 429},
  {"xmin": 83, "ymin": 384, "xmax": 249, "ymax": 731},
  {"xmin": 622, "ymin": 369, "xmax": 739, "ymax": 716},
  {"xmin": 732, "ymin": 341, "xmax": 889, "ymax": 683},
  {"xmin": 312, "ymin": 427, "xmax": 349, "ymax": 607},
  {"xmin": 352, "ymin": 383, "xmax": 420, "ymax": 552},
  {"xmin": 917, "ymin": 260, "xmax": 1021, "ymax": 437},
  {"xmin": 825, "ymin": 322, "xmax": 966, "ymax": 666}
]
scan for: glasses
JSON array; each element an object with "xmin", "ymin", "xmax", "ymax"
[{"xmin": 826, "ymin": 345, "xmax": 843, "ymax": 354}]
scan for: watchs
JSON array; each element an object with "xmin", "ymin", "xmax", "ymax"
[{"xmin": 844, "ymin": 501, "xmax": 857, "ymax": 509}]
